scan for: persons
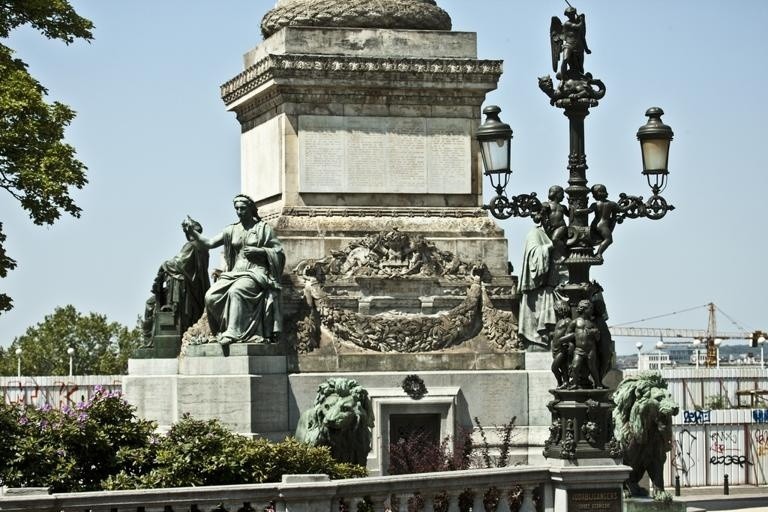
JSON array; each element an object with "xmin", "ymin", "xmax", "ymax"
[
  {"xmin": 550, "ymin": 298, "xmax": 603, "ymax": 391},
  {"xmin": 575, "ymin": 184, "xmax": 619, "ymax": 259},
  {"xmin": 143, "ymin": 216, "xmax": 209, "ymax": 326},
  {"xmin": 184, "ymin": 194, "xmax": 284, "ymax": 337},
  {"xmin": 533, "ymin": 185, "xmax": 570, "ymax": 256},
  {"xmin": 560, "ymin": 6, "xmax": 591, "ymax": 89}
]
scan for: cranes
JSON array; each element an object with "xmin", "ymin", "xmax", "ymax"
[{"xmin": 607, "ymin": 302, "xmax": 767, "ymax": 367}]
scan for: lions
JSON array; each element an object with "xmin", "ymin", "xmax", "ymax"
[
  {"xmin": 295, "ymin": 380, "xmax": 376, "ymax": 473},
  {"xmin": 608, "ymin": 370, "xmax": 680, "ymax": 507}
]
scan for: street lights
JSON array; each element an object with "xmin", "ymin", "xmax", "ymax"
[
  {"xmin": 655, "ymin": 341, "xmax": 664, "ymax": 369},
  {"xmin": 712, "ymin": 338, "xmax": 721, "ymax": 367},
  {"xmin": 692, "ymin": 339, "xmax": 700, "ymax": 369},
  {"xmin": 756, "ymin": 335, "xmax": 765, "ymax": 368},
  {"xmin": 67, "ymin": 347, "xmax": 75, "ymax": 375},
  {"xmin": 634, "ymin": 341, "xmax": 643, "ymax": 371},
  {"xmin": 15, "ymin": 348, "xmax": 23, "ymax": 377},
  {"xmin": 475, "ymin": 2, "xmax": 676, "ymax": 461}
]
